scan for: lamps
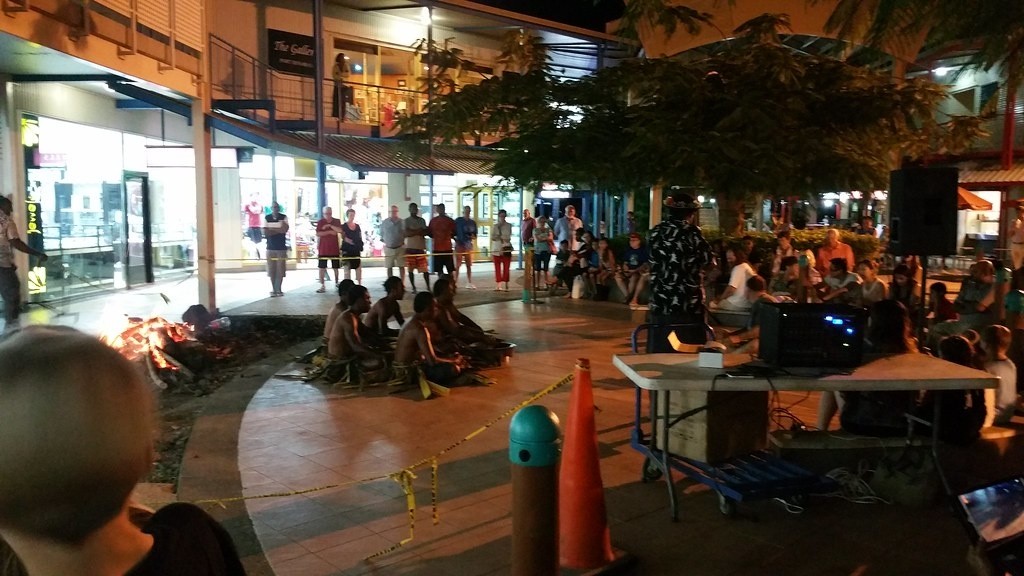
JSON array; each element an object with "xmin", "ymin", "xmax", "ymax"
[{"xmin": 949, "ymin": 474, "xmax": 1024, "ymax": 576}]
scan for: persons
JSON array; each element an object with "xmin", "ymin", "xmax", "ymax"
[
  {"xmin": 0, "ymin": 195, "xmax": 48, "ymax": 327},
  {"xmin": 341, "ymin": 209, "xmax": 363, "ymax": 285},
  {"xmin": 452, "ymin": 206, "xmax": 478, "ymax": 290},
  {"xmin": 772, "ymin": 195, "xmax": 810, "ymax": 229},
  {"xmin": 323, "ymin": 274, "xmax": 504, "ymax": 384},
  {"xmin": 709, "ymin": 248, "xmax": 757, "ymax": 310},
  {"xmin": 554, "ymin": 205, "xmax": 583, "ymax": 250},
  {"xmin": 614, "ymin": 233, "xmax": 650, "ymax": 306},
  {"xmin": 545, "ymin": 227, "xmax": 614, "ymax": 307},
  {"xmin": 380, "ymin": 203, "xmax": 456, "ymax": 289},
  {"xmin": 521, "ymin": 209, "xmax": 553, "ymax": 291},
  {"xmin": 333, "ymin": 53, "xmax": 349, "ymax": 118},
  {"xmin": 712, "ymin": 219, "xmax": 1024, "ymax": 442},
  {"xmin": 491, "ymin": 210, "xmax": 513, "ymax": 291},
  {"xmin": 316, "ymin": 207, "xmax": 342, "ymax": 293},
  {"xmin": 646, "ymin": 192, "xmax": 712, "ymax": 353},
  {"xmin": 263, "ymin": 201, "xmax": 289, "ymax": 297},
  {"xmin": 1008, "ymin": 206, "xmax": 1024, "ymax": 269},
  {"xmin": 626, "ymin": 212, "xmax": 635, "ymax": 232},
  {"xmin": 0, "ymin": 323, "xmax": 246, "ymax": 576},
  {"xmin": 244, "ymin": 192, "xmax": 263, "ymax": 244}
]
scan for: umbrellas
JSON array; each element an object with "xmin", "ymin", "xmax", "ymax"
[{"xmin": 956, "ymin": 187, "xmax": 992, "ymax": 210}]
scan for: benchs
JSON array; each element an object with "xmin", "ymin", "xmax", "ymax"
[
  {"xmin": 768, "ymin": 413, "xmax": 1024, "ymax": 450},
  {"xmin": 630, "ymin": 303, "xmax": 751, "ymax": 324}
]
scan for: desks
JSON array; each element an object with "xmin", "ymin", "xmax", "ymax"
[{"xmin": 611, "ymin": 352, "xmax": 1003, "ymax": 520}]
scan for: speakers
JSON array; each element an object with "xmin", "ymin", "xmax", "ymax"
[{"xmin": 887, "ymin": 167, "xmax": 960, "ymax": 255}]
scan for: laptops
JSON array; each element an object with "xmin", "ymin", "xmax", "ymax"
[{"xmin": 951, "ymin": 474, "xmax": 1024, "ymax": 576}]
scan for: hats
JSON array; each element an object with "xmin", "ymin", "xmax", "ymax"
[
  {"xmin": 626, "ymin": 231, "xmax": 642, "ymax": 239},
  {"xmin": 663, "ymin": 192, "xmax": 702, "ymax": 209}
]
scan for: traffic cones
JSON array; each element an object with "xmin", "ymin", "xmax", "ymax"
[{"xmin": 542, "ymin": 358, "xmax": 632, "ymax": 575}]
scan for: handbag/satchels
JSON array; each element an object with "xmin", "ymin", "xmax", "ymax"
[{"xmin": 502, "ymin": 245, "xmax": 512, "ymax": 256}]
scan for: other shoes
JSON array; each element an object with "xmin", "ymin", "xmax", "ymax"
[
  {"xmin": 271, "ymin": 290, "xmax": 284, "ymax": 298},
  {"xmin": 317, "ymin": 287, "xmax": 325, "ymax": 293},
  {"xmin": 624, "ymin": 296, "xmax": 638, "ymax": 306},
  {"xmin": 494, "ymin": 286, "xmax": 510, "ymax": 292}
]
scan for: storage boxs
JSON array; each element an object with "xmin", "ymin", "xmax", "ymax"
[{"xmin": 655, "ymin": 389, "xmax": 769, "ymax": 463}]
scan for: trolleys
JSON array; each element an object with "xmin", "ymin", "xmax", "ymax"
[{"xmin": 630, "ymin": 320, "xmax": 839, "ymax": 523}]
